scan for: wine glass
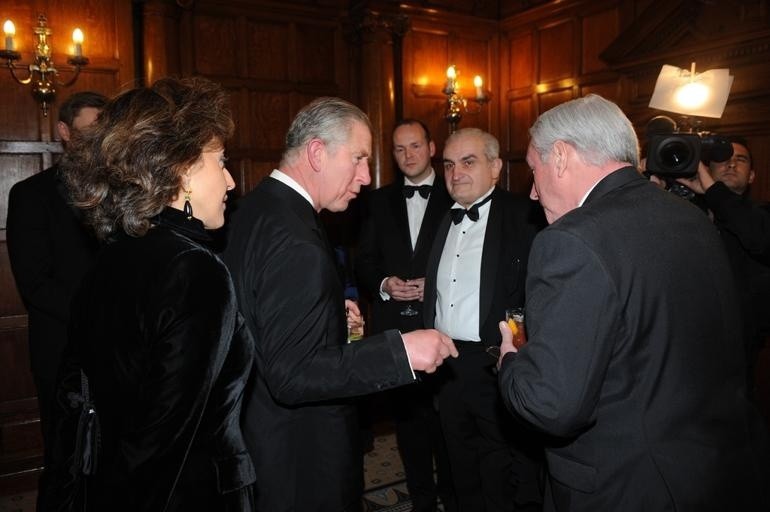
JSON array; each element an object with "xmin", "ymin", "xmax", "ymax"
[{"xmin": 394, "ymin": 277, "xmax": 419, "ymax": 317}]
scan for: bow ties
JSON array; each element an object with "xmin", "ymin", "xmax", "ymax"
[
  {"xmin": 450, "ymin": 204, "xmax": 481, "ymax": 225},
  {"xmin": 404, "ymin": 184, "xmax": 432, "ymax": 199}
]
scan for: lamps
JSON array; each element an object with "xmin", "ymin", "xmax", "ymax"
[
  {"xmin": 442, "ymin": 65, "xmax": 489, "ymax": 136},
  {"xmin": 0, "ymin": 15, "xmax": 89, "ymax": 118}
]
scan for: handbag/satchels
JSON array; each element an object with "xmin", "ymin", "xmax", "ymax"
[{"xmin": 63, "ymin": 401, "xmax": 104, "ymax": 477}]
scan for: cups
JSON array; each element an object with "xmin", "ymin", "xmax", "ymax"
[
  {"xmin": 348, "ymin": 313, "xmax": 365, "ymax": 341},
  {"xmin": 504, "ymin": 309, "xmax": 525, "ymax": 354}
]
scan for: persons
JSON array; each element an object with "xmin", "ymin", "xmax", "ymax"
[
  {"xmin": 44, "ymin": 77, "xmax": 257, "ymax": 512},
  {"xmin": 676, "ymin": 134, "xmax": 770, "ymax": 402},
  {"xmin": 7, "ymin": 93, "xmax": 111, "ymax": 444},
  {"xmin": 360, "ymin": 120, "xmax": 459, "ymax": 512},
  {"xmin": 495, "ymin": 93, "xmax": 769, "ymax": 512},
  {"xmin": 223, "ymin": 96, "xmax": 459, "ymax": 512},
  {"xmin": 423, "ymin": 126, "xmax": 548, "ymax": 512}
]
